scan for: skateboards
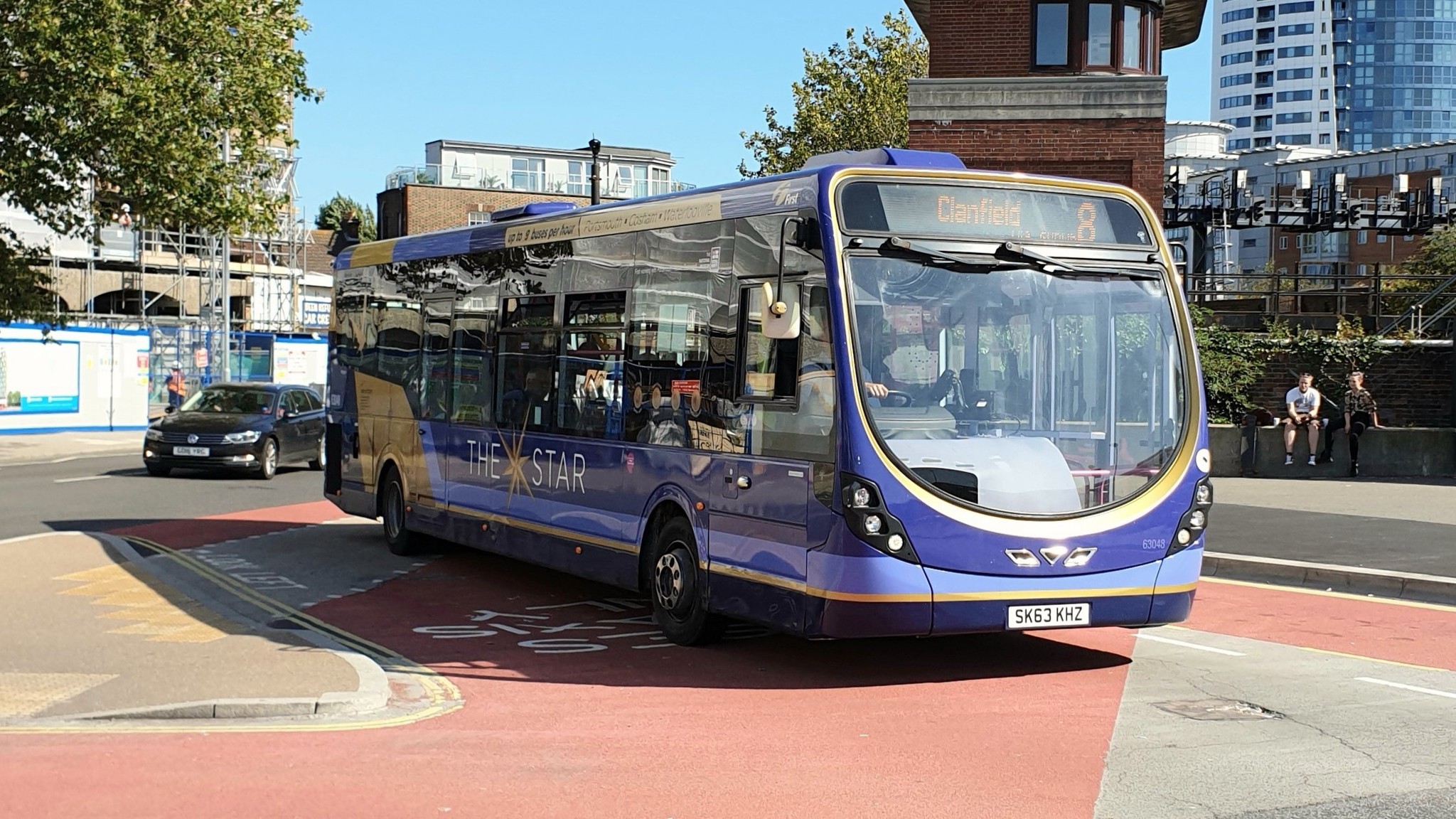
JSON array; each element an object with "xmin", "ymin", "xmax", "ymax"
[{"xmin": 1239, "ymin": 414, "xmax": 1261, "ymax": 478}]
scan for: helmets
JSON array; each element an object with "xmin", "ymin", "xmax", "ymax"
[{"xmin": 170, "ymin": 360, "xmax": 182, "ymax": 368}]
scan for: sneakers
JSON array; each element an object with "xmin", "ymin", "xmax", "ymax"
[
  {"xmin": 1307, "ymin": 456, "xmax": 1316, "ymax": 465},
  {"xmin": 1285, "ymin": 454, "xmax": 1294, "ymax": 465}
]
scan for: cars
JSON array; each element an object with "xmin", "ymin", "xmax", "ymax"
[{"xmin": 143, "ymin": 381, "xmax": 327, "ymax": 481}]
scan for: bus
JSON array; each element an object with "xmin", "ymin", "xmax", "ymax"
[{"xmin": 323, "ymin": 146, "xmax": 1215, "ymax": 652}]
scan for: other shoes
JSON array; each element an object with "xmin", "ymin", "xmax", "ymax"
[
  {"xmin": 1316, "ymin": 451, "xmax": 1334, "ymax": 463},
  {"xmin": 1351, "ymin": 460, "xmax": 1359, "ymax": 477}
]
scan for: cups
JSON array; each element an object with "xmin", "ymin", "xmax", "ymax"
[
  {"xmin": 1274, "ymin": 417, "xmax": 1280, "ymax": 428},
  {"xmin": 1322, "ymin": 419, "xmax": 1329, "ymax": 427}
]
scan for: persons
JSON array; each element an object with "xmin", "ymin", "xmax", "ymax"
[
  {"xmin": 808, "ymin": 315, "xmax": 956, "ymax": 406},
  {"xmin": 1315, "ymin": 373, "xmax": 1385, "ymax": 477},
  {"xmin": 209, "ymin": 391, "xmax": 286, "ymax": 418},
  {"xmin": 1283, "ymin": 375, "xmax": 1321, "ymax": 465},
  {"xmin": 165, "ymin": 363, "xmax": 187, "ymax": 412}
]
